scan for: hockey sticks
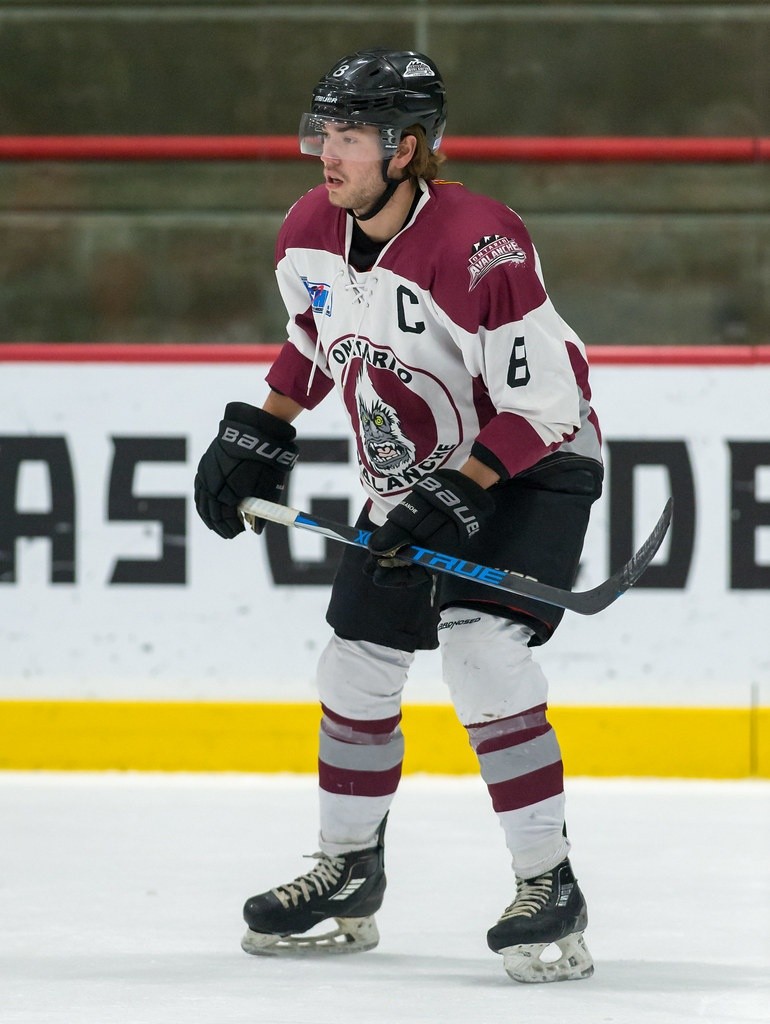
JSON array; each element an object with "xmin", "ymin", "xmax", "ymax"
[{"xmin": 239, "ymin": 494, "xmax": 675, "ymax": 616}]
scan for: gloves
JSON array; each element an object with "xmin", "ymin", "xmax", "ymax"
[
  {"xmin": 196, "ymin": 402, "xmax": 299, "ymax": 537},
  {"xmin": 372, "ymin": 467, "xmax": 485, "ymax": 573}
]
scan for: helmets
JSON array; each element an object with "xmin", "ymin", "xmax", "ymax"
[{"xmin": 312, "ymin": 50, "xmax": 446, "ymax": 135}]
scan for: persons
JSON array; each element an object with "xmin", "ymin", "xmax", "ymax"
[{"xmin": 194, "ymin": 42, "xmax": 605, "ymax": 982}]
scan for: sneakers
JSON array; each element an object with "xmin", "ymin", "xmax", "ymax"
[
  {"xmin": 242, "ymin": 844, "xmax": 389, "ymax": 954},
  {"xmin": 488, "ymin": 862, "xmax": 596, "ymax": 981}
]
